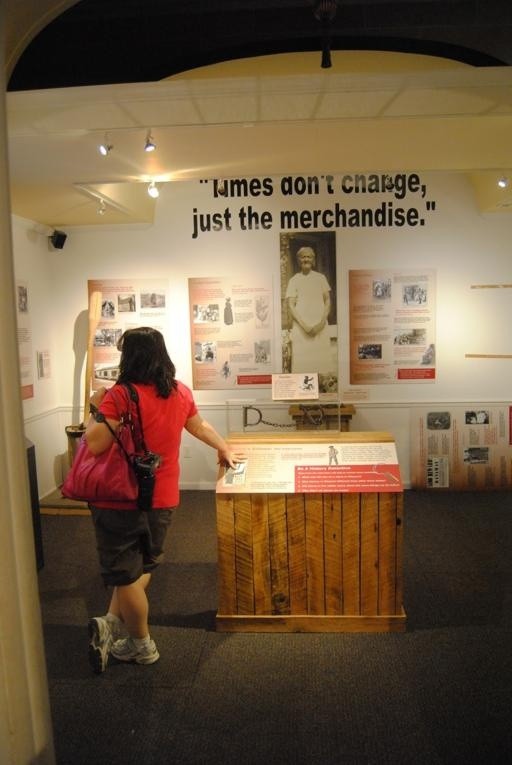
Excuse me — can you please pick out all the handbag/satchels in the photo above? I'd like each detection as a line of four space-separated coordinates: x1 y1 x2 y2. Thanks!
61 424 139 502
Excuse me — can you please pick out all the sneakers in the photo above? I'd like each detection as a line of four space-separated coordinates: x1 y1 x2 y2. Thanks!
111 636 160 665
87 616 114 674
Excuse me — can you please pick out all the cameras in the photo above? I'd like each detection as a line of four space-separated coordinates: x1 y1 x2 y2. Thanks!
134 452 160 513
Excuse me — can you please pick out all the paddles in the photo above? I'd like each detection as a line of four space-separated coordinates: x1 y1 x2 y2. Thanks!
83 291 103 428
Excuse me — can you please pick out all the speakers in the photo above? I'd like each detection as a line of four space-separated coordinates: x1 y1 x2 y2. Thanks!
49 229 67 248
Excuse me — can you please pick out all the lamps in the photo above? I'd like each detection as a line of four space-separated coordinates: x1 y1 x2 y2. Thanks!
149 181 160 198
98 132 113 157
143 129 156 151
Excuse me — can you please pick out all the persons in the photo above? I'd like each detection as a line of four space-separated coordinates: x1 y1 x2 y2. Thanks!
225 462 244 483
417 287 426 304
327 446 339 465
284 245 334 374
76 327 247 673
206 347 213 360
403 287 409 304
463 448 489 464
224 360 230 378
